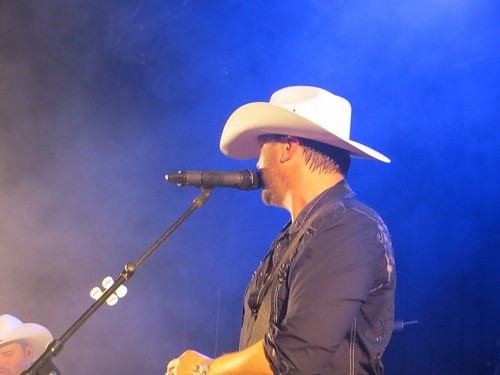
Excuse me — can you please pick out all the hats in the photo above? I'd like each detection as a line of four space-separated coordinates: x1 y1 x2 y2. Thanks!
0 314 55 363
219 83 391 167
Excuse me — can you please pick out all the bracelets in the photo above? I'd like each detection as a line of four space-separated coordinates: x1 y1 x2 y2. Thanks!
192 359 215 375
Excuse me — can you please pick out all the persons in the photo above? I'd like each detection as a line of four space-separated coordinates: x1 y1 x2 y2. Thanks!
162 85 397 375
0 314 54 375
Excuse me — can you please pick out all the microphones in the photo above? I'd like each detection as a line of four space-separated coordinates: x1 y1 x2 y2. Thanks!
164 169 264 190
